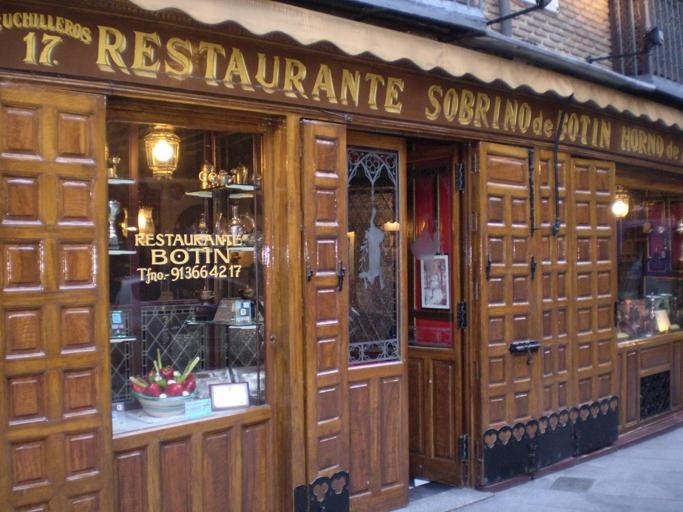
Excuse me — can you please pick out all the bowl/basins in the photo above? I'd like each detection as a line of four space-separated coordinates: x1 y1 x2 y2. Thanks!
131 387 199 419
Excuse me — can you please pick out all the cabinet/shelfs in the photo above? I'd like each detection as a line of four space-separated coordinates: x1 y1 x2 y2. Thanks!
107 178 136 344
185 183 265 371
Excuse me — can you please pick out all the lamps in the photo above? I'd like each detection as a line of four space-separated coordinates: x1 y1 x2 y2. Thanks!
122 205 155 243
586 27 666 64
616 184 630 218
488 1 551 27
143 125 180 181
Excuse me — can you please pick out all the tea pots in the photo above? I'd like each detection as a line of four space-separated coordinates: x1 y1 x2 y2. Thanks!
194 287 216 307
198 159 249 191
238 286 257 300
